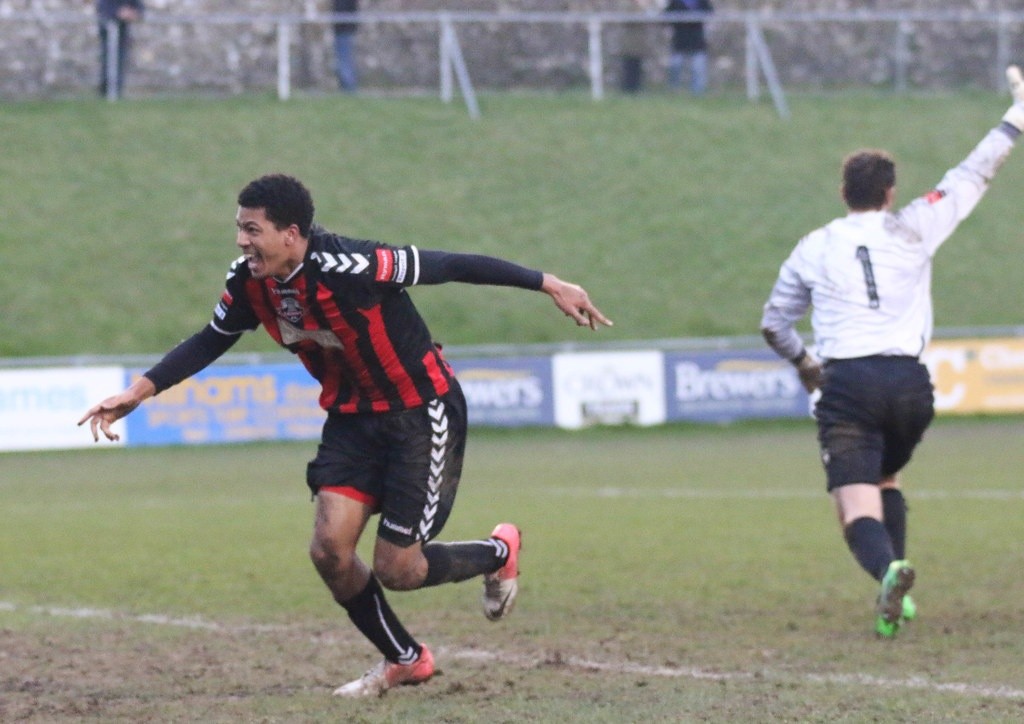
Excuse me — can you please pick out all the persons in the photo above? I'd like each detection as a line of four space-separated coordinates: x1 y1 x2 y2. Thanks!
79 173 613 698
757 65 1023 636
98 0 714 101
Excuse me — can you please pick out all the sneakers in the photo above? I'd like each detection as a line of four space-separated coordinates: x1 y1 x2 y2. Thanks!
332 642 434 699
875 593 916 622
481 523 522 621
873 559 916 640
